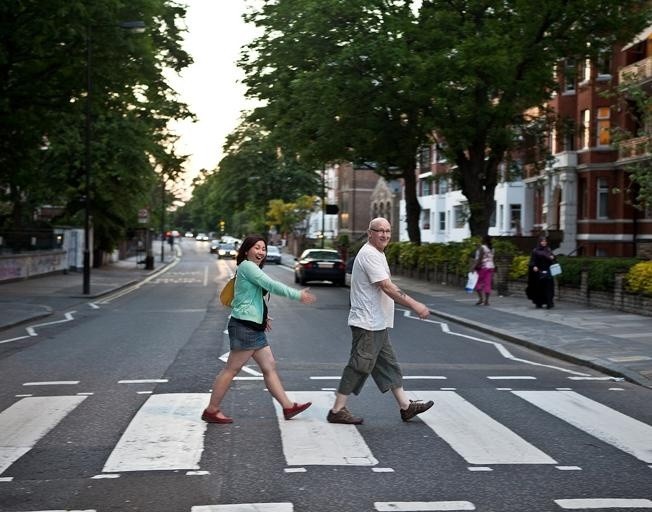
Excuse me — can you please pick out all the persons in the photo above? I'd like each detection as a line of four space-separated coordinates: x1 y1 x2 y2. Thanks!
327 216 435 424
201 236 317 424
525 236 557 308
470 235 495 306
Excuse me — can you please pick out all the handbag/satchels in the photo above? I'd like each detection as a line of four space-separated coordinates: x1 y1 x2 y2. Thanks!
549 263 562 277
219 277 236 308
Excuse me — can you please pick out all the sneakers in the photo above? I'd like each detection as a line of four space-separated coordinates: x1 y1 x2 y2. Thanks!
400 400 434 421
326 406 364 424
535 303 555 309
476 298 491 306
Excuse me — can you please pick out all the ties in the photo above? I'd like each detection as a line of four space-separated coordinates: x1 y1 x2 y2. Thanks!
283 402 312 420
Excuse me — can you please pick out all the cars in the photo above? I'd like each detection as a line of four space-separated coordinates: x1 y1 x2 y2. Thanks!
293 248 346 288
166 230 244 260
266 246 281 264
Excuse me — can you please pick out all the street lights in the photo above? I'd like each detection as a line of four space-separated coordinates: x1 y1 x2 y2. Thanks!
159 167 179 262
81 17 148 295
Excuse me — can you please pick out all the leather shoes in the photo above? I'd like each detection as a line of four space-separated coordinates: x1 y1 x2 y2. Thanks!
201 409 233 424
